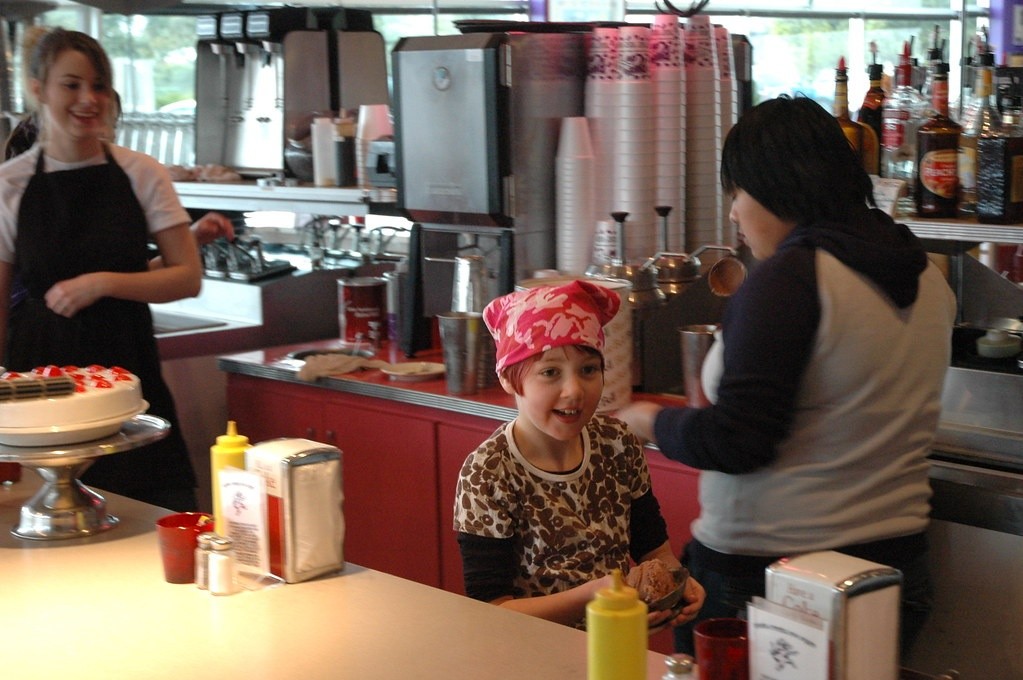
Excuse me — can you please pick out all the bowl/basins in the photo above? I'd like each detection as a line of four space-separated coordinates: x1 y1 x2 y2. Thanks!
647 568 689 629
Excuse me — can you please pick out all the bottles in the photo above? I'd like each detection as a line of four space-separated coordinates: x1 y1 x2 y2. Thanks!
585 567 647 680
209 420 253 537
309 106 356 187
828 0 1023 228
661 653 699 680
208 538 233 596
193 533 218 589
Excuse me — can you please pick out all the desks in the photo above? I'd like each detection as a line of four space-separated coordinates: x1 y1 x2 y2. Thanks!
0 479 699 680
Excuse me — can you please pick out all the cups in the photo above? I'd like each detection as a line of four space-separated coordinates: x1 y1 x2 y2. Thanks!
433 253 496 394
511 11 754 279
692 618 750 680
678 325 717 410
357 103 397 188
155 512 213 583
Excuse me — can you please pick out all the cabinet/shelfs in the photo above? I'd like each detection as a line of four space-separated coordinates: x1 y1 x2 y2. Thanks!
226 370 698 656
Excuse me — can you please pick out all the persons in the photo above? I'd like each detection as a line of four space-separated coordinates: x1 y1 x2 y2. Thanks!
618 92 960 659
1 26 233 513
449 280 706 635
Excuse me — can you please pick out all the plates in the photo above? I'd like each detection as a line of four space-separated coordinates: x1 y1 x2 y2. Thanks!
378 361 445 382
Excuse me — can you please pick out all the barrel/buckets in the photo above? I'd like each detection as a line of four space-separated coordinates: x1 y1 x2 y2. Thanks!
336 271 410 348
512 275 634 412
